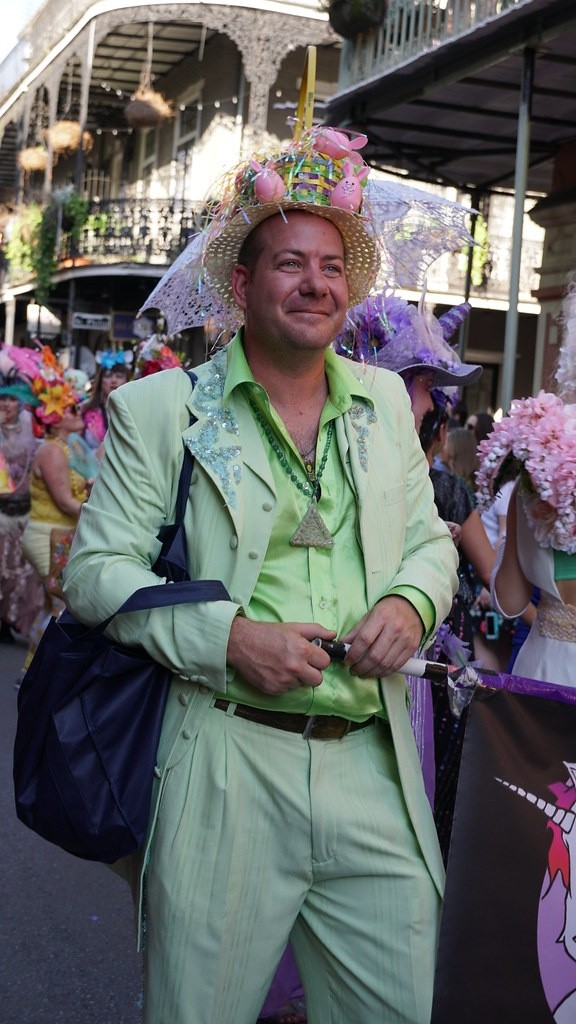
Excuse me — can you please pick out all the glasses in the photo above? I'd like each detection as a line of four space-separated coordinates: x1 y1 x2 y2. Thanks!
64 404 82 417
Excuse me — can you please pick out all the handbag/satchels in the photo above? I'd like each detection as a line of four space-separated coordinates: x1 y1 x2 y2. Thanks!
10 564 170 866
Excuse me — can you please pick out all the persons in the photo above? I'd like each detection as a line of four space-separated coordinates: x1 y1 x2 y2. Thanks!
1 192 575 1023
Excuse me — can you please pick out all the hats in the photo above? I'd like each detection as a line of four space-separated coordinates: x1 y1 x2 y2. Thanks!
207 151 380 312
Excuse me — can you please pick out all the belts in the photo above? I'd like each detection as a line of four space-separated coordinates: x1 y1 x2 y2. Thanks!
213 699 382 740
